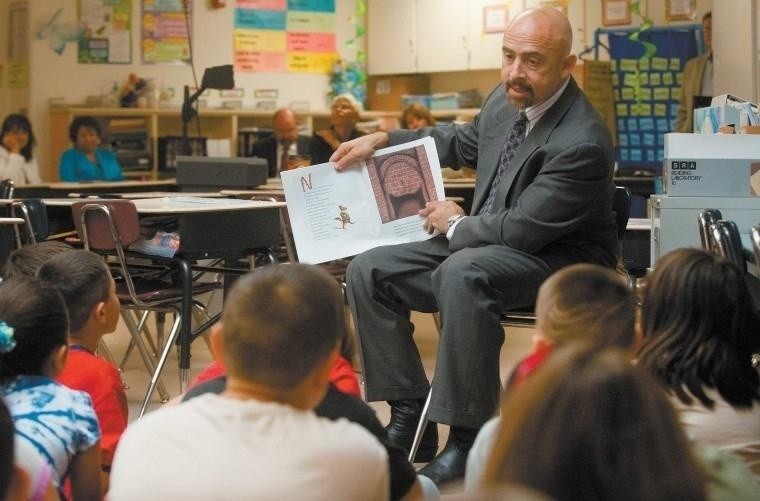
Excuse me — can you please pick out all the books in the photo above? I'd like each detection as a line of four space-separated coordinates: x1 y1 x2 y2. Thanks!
280 136 446 265
400 88 482 111
101 118 310 171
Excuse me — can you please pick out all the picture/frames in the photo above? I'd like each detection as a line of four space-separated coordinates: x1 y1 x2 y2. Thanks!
664 0 696 22
485 6 508 33
601 0 632 26
540 1 568 17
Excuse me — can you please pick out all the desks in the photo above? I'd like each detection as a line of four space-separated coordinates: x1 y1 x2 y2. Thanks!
609 173 662 198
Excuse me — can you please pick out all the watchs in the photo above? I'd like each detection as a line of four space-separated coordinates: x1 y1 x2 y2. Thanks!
445 214 464 235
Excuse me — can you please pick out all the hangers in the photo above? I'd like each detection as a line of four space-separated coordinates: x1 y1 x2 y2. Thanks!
577 27 618 62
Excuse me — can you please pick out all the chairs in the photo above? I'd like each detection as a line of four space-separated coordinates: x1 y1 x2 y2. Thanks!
698 223 759 279
699 208 722 252
407 186 632 466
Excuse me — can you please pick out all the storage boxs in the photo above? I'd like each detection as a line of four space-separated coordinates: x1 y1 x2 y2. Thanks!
663 131 759 197
426 96 482 109
401 94 430 111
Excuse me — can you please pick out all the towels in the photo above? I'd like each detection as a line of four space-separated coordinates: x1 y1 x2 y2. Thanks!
583 58 618 148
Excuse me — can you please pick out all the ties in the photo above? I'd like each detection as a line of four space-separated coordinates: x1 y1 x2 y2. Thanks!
281 144 289 171
474 113 526 215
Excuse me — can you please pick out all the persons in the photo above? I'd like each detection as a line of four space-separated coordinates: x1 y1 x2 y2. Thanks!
311 94 370 165
0 114 40 186
102 261 441 501
0 242 129 501
328 6 622 491
466 247 760 501
400 103 436 130
60 116 124 181
252 109 310 178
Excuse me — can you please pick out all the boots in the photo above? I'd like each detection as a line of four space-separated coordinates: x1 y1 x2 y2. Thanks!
384 399 438 463
417 425 479 485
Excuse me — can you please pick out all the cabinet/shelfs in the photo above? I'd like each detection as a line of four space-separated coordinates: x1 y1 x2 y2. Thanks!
48 100 479 184
366 68 503 111
646 194 759 278
